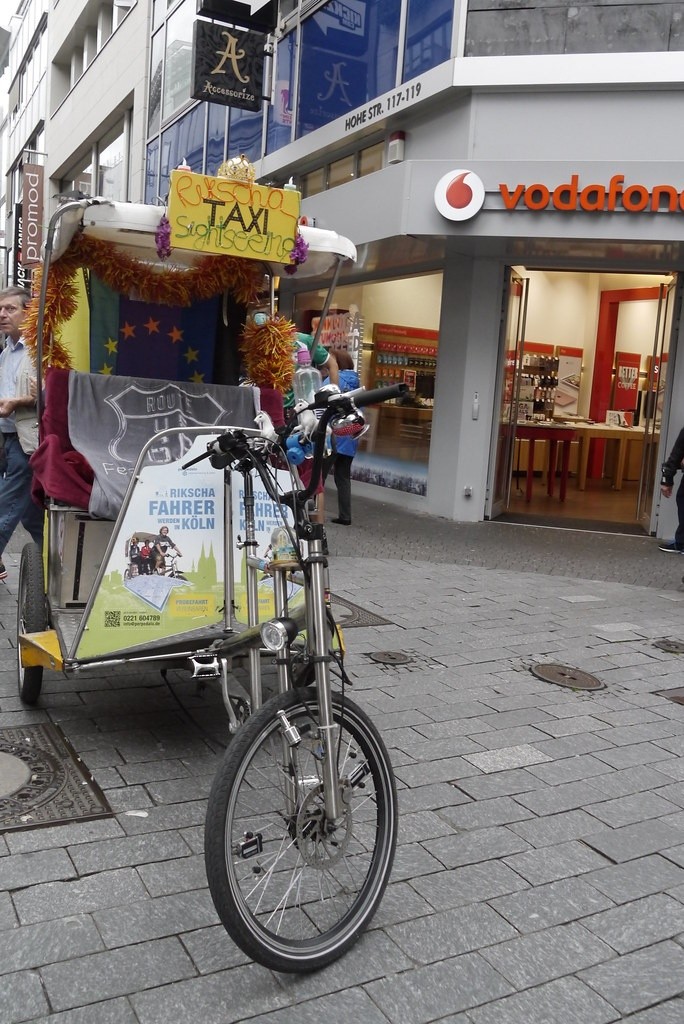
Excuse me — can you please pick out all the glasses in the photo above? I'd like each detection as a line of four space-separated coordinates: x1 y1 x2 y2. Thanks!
0 305 25 314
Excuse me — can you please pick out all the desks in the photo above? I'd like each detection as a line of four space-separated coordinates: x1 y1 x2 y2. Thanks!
498 419 660 505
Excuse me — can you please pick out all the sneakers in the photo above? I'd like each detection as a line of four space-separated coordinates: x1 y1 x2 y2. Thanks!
658 544 684 553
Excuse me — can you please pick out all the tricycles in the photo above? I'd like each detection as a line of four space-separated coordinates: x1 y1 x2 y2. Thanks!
18 198 409 974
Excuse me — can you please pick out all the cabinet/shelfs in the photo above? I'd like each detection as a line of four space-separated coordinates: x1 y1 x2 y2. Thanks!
519 345 562 420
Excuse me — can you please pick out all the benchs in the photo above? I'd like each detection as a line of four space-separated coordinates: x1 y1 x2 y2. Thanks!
34 363 291 518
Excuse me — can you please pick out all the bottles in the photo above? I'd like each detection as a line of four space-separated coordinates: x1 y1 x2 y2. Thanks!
293 349 322 405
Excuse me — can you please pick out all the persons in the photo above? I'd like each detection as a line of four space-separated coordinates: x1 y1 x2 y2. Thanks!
129 526 183 579
0 287 48 579
245 310 361 526
659 426 684 554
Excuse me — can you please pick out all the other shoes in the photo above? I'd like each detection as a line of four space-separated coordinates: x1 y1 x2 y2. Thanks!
0 565 7 578
332 519 352 526
